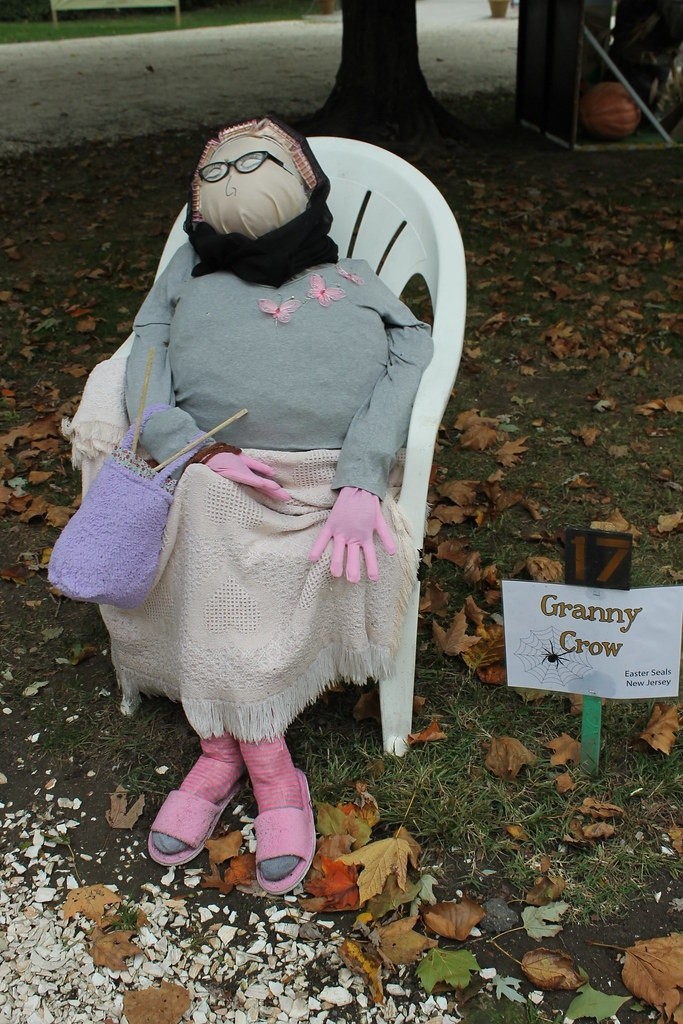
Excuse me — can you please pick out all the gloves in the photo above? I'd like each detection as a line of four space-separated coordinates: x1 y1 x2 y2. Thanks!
198 447 291 501
314 487 396 583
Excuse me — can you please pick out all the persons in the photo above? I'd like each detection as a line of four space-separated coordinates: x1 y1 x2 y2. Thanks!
119 113 433 894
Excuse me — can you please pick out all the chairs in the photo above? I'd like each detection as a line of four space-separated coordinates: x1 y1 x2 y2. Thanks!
62 135 468 756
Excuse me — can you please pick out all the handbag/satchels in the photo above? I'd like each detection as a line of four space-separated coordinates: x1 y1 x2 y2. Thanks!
47 403 213 611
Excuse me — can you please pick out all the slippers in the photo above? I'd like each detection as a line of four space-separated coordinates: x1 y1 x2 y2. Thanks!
147 768 249 866
252 768 316 895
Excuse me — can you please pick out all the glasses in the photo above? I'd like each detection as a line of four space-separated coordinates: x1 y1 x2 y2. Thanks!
197 151 304 185
